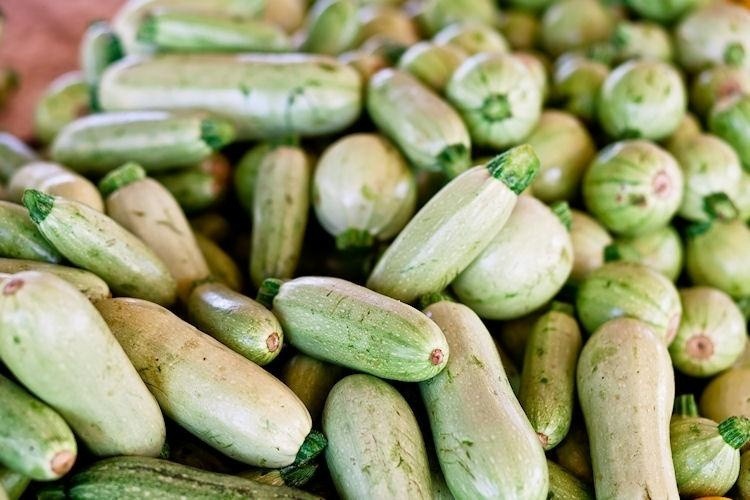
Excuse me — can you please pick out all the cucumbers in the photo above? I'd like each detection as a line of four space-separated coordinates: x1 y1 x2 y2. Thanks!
1 0 750 500
